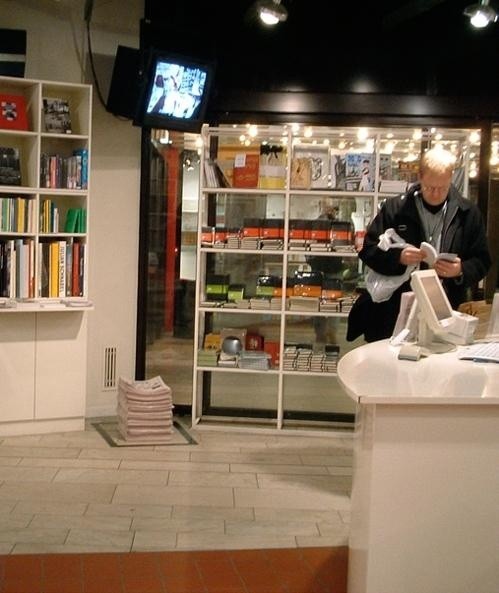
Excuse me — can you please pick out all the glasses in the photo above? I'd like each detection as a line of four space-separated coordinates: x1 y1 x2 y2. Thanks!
422 185 448 194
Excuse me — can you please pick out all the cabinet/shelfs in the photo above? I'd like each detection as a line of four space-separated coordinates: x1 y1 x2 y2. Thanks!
191 122 482 427
1 70 98 435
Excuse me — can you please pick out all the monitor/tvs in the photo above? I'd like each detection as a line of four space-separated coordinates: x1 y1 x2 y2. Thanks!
106 44 221 133
410 269 456 355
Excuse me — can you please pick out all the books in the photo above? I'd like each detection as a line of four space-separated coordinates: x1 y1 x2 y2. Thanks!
195 137 463 372
114 372 174 441
1 88 89 309
459 339 499 363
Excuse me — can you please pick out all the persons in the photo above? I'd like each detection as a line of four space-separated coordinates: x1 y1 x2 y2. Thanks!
359 145 493 341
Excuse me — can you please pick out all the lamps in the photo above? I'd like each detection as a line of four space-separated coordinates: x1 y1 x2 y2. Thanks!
259 0 288 35
464 0 498 33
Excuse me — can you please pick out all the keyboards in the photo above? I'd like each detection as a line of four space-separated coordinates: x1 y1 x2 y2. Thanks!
458 343 499 363
